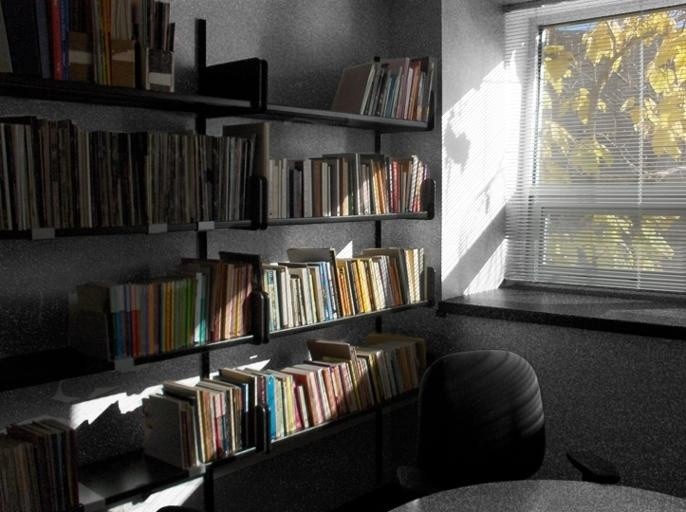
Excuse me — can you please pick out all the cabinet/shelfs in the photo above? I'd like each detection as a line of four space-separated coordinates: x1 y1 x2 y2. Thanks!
0 2 442 512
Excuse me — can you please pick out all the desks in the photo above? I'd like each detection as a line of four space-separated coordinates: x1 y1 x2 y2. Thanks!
387 479 686 511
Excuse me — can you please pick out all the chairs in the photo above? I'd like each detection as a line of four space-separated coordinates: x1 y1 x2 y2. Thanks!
419 350 624 485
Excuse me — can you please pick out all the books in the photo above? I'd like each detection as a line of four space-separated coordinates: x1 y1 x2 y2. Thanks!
142 368 259 471
0 0 176 91
236 336 432 442
0 117 253 230
76 262 256 361
218 245 429 332
222 122 430 219
331 55 432 122
0 419 81 512
